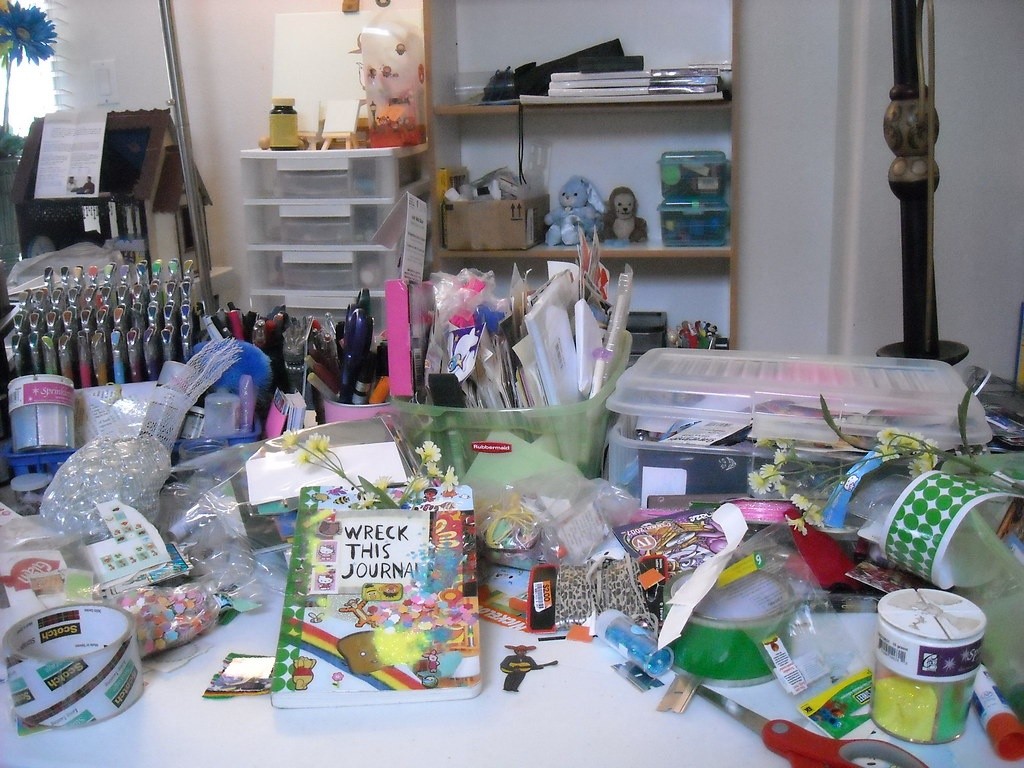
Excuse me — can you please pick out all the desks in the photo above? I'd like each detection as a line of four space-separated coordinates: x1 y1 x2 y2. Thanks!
0 552 1024 768
192 265 235 310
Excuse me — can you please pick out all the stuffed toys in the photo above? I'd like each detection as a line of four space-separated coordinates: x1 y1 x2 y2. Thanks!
544 175 606 247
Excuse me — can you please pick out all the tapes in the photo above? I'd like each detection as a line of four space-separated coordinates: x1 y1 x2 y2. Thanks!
658 566 794 688
1 603 144 731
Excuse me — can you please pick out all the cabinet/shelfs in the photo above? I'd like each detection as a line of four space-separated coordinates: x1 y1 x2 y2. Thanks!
241 138 430 336
423 0 739 348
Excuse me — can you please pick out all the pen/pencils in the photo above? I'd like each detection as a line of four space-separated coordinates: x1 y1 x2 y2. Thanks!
199 298 292 364
304 288 391 409
10 258 196 386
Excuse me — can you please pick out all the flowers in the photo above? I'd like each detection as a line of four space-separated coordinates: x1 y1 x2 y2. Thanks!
280 425 460 510
0 0 58 156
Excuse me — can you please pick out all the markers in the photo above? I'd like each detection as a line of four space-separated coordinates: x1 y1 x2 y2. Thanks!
971 661 1024 762
339 310 374 405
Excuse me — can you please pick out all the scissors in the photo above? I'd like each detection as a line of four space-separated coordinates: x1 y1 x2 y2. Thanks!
696 685 930 768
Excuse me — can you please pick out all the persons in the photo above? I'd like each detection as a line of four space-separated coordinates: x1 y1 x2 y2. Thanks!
595 186 648 243
67 176 95 194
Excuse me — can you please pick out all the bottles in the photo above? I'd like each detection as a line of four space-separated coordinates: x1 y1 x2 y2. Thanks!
269 98 299 151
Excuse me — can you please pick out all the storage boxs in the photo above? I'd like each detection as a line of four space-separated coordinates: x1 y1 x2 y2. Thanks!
604 347 992 499
393 329 632 479
444 194 550 251
656 150 726 204
656 201 731 247
